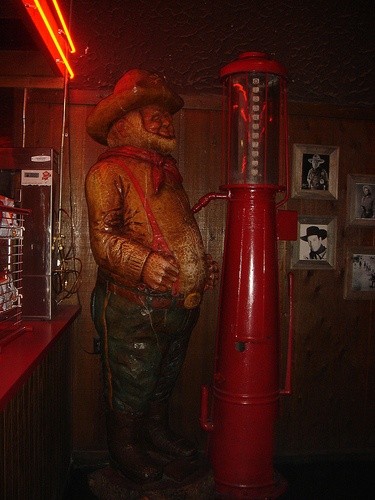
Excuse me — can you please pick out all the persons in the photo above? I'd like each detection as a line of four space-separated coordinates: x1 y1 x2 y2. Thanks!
300 226 328 261
85 69 219 483
360 186 374 218
308 154 329 192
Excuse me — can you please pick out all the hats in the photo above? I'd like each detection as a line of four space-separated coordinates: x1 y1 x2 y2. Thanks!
300 226 327 242
308 154 325 164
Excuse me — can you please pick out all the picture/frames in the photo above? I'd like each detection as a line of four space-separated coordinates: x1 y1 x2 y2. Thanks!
345 173 375 229
342 246 375 302
291 215 337 270
292 143 340 200
